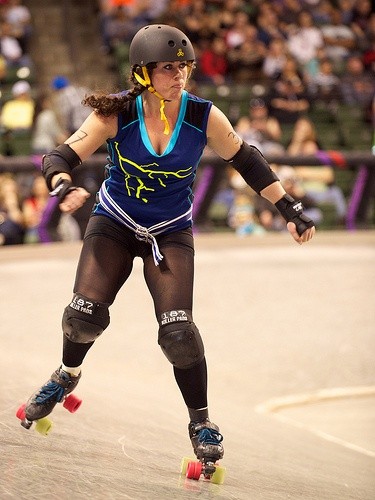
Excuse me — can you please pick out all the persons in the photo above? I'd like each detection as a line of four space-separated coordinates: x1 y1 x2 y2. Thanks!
1 0 375 248
15 24 316 486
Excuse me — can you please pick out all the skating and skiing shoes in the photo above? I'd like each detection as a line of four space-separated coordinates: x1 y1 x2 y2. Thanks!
178 419 225 486
14 366 85 435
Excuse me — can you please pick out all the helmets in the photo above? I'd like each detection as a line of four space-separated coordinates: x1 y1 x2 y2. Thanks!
129 24 197 67
